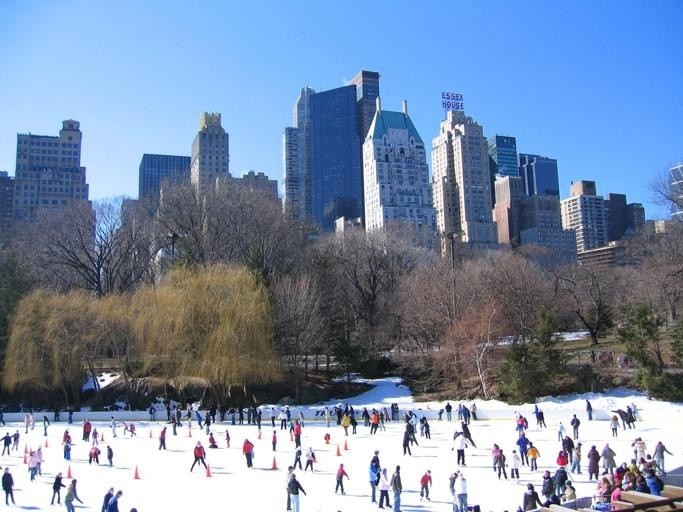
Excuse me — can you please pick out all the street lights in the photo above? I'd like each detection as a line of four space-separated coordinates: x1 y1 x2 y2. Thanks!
434 229 469 390
163 231 189 261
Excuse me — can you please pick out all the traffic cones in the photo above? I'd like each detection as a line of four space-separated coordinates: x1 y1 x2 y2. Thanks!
289 433 294 441
271 456 277 470
335 443 341 456
257 432 261 439
343 439 349 450
187 430 191 437
21 433 142 480
149 430 153 437
325 434 330 444
312 455 316 462
205 463 211 477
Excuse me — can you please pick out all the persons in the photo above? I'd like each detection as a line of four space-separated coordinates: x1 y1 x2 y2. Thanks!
587 346 631 371
490 399 673 511
362 401 481 511
123 420 137 438
1 406 122 512
266 403 358 511
147 403 263 473
